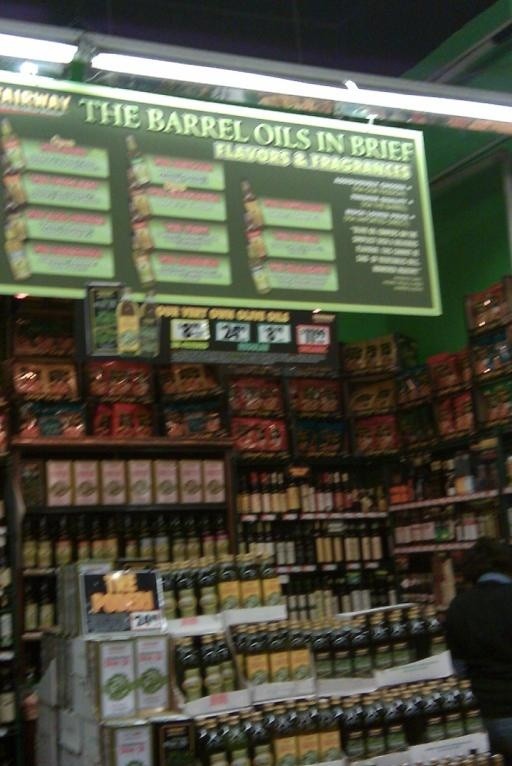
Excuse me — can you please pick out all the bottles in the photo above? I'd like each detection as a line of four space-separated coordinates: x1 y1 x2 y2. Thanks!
0 436 512 766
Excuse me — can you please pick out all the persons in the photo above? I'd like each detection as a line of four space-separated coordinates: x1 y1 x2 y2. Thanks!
446 536 511 761
